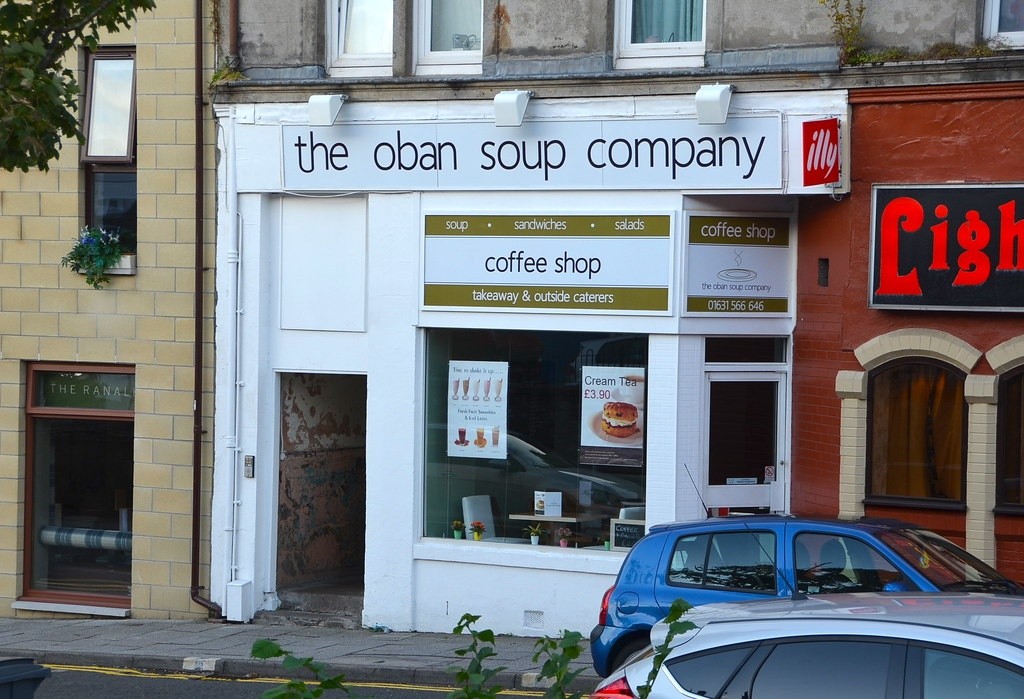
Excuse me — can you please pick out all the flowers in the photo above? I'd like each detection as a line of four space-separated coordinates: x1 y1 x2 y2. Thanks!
555 526 573 539
469 521 486 537
450 520 466 532
62 222 122 289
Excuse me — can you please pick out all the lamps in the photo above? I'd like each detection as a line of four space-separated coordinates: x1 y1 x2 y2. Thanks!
695 81 736 125
494 88 535 128
307 93 350 128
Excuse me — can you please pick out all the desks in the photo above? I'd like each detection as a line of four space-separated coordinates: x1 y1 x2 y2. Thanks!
508 512 586 546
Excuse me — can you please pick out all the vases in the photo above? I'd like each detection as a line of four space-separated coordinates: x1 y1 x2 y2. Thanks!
454 529 463 540
559 538 568 548
473 532 482 541
104 253 137 270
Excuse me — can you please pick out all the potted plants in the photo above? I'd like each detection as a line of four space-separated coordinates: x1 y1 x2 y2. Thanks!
521 521 547 545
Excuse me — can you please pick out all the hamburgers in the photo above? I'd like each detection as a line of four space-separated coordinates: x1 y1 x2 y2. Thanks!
601 402 641 438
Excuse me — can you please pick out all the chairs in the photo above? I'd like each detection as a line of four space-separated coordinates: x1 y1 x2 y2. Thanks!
583 507 646 551
461 494 532 544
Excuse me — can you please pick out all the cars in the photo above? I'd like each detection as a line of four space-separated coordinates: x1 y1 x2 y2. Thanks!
590 588 1024 699
427 421 646 546
589 461 1024 679
